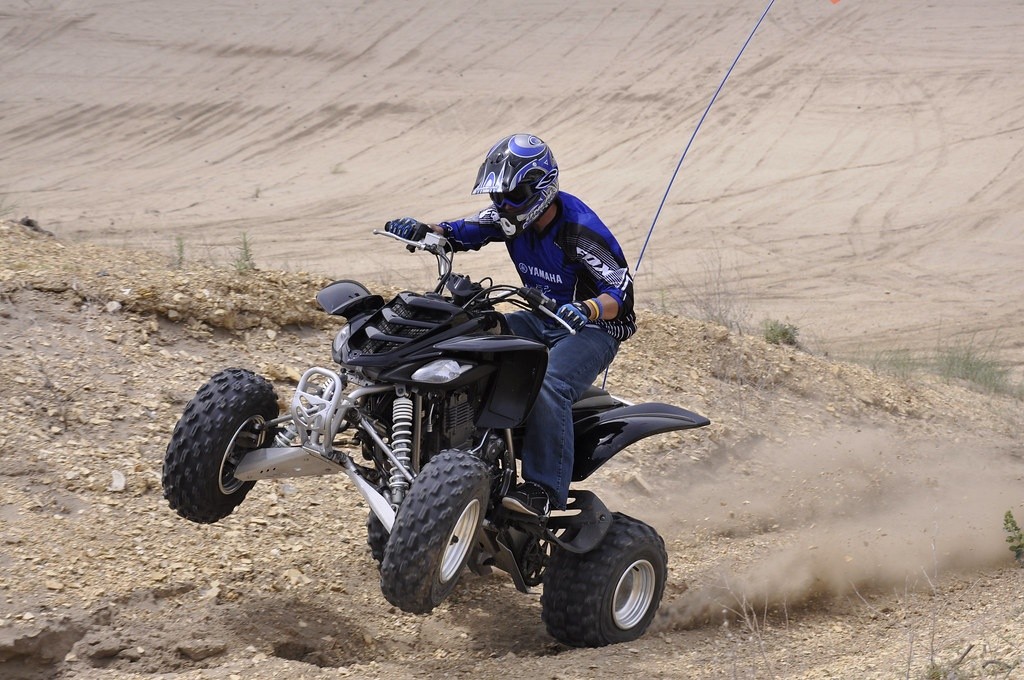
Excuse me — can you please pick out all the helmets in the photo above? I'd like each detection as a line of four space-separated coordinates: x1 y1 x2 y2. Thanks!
471 131 559 240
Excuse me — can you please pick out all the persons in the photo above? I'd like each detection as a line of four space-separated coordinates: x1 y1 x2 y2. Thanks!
384 134 638 517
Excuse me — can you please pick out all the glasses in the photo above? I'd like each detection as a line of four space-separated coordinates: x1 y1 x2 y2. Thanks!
489 178 543 208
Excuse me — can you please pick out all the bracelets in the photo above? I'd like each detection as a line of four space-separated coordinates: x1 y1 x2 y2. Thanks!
588 298 602 320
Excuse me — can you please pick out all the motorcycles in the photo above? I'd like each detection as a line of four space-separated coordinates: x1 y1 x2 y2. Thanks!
162 222 711 646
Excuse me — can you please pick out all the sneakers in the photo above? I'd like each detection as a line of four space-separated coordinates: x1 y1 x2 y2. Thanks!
501 481 551 517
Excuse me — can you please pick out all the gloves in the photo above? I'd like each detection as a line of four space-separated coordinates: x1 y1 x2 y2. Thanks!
555 301 590 335
385 217 431 242
